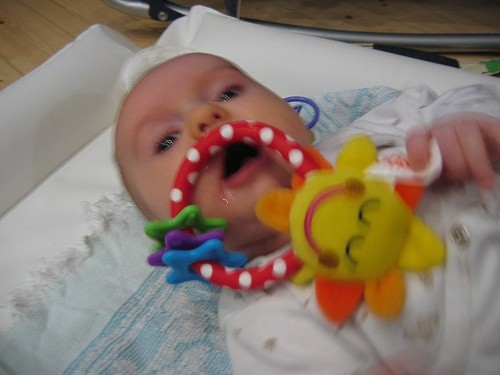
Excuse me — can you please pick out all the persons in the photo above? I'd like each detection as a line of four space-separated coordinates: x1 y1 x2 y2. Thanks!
114 51 499 375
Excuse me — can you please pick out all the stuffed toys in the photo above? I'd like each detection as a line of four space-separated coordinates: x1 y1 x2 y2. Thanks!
254 133 447 325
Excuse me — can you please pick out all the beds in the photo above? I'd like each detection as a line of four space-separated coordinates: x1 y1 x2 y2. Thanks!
0 4 500 375
